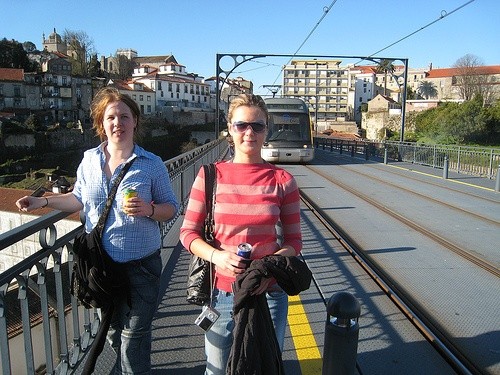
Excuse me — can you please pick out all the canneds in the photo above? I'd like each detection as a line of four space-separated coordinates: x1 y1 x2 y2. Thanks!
237 243 252 269
123 187 138 213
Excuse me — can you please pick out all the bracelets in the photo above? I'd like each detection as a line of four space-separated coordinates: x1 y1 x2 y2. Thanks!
42 197 48 208
146 201 155 218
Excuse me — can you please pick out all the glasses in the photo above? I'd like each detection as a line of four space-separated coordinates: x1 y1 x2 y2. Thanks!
229 121 269 135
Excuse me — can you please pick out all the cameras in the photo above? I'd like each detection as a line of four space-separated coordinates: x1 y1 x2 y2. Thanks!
194 306 220 332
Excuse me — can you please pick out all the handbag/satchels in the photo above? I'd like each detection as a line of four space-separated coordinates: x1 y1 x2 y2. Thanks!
69 229 118 310
185 231 217 306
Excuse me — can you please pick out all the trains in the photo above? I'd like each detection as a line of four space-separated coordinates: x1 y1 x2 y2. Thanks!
261 97 314 163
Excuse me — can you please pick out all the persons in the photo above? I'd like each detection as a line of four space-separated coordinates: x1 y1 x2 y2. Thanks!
15 88 179 375
179 93 302 375
